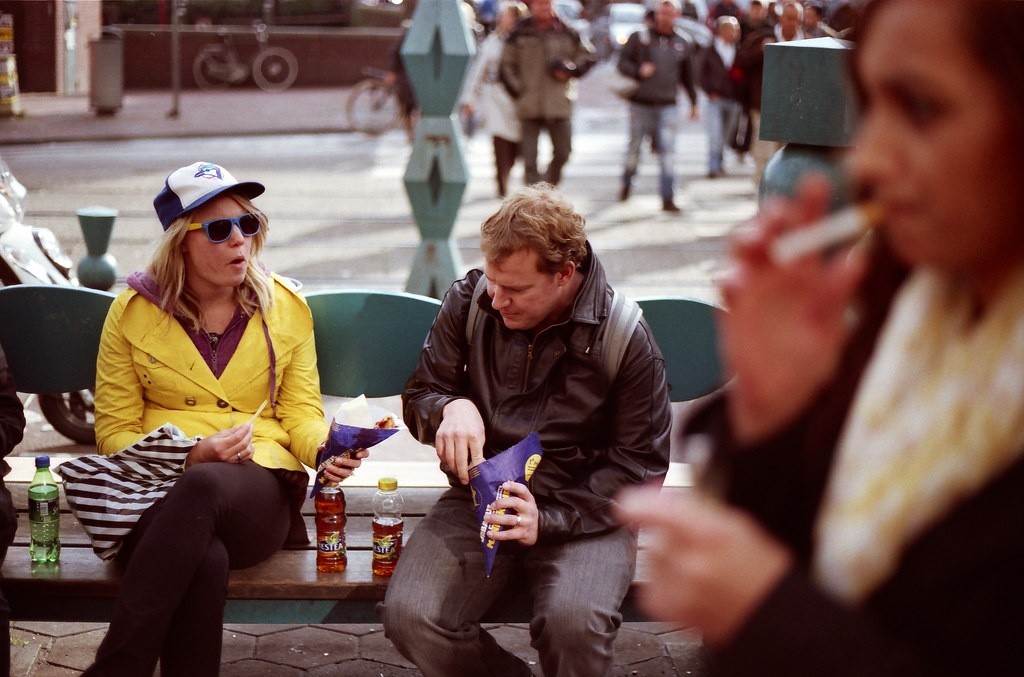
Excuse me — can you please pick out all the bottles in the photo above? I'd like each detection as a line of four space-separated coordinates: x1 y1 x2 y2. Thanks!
371 477 405 578
27 455 61 564
314 481 347 574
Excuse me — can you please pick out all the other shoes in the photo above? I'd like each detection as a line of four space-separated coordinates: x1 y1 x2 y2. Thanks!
620 175 632 199
663 202 679 212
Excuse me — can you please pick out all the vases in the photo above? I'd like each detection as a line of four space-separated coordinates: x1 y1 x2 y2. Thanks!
76 205 120 288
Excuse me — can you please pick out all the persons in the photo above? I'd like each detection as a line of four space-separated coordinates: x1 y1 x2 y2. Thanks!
79 163 368 677
0 344 27 677
613 0 1024 677
384 0 853 213
381 181 673 677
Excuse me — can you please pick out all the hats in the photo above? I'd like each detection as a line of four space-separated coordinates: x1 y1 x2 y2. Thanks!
154 161 266 232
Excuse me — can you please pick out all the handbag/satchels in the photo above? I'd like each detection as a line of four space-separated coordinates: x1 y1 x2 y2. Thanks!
52 422 203 561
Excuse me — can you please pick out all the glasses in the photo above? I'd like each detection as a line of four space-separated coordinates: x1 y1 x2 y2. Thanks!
186 211 261 242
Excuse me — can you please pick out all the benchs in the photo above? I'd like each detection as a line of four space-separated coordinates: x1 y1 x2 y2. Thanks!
0 265 731 623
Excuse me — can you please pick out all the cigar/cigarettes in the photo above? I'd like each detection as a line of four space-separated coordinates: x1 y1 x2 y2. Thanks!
772 197 890 269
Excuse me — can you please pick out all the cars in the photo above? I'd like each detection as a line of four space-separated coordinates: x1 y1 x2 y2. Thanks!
591 2 650 57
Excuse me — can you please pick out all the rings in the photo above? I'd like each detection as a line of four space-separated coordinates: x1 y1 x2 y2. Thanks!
515 515 520 527
237 452 242 460
346 469 353 477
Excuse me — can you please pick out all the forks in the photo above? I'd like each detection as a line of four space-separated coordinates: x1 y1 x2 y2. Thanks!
249 399 268 424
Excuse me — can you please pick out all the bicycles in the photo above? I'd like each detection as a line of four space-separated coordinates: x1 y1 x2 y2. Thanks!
0 159 97 447
192 17 299 94
340 63 479 140
